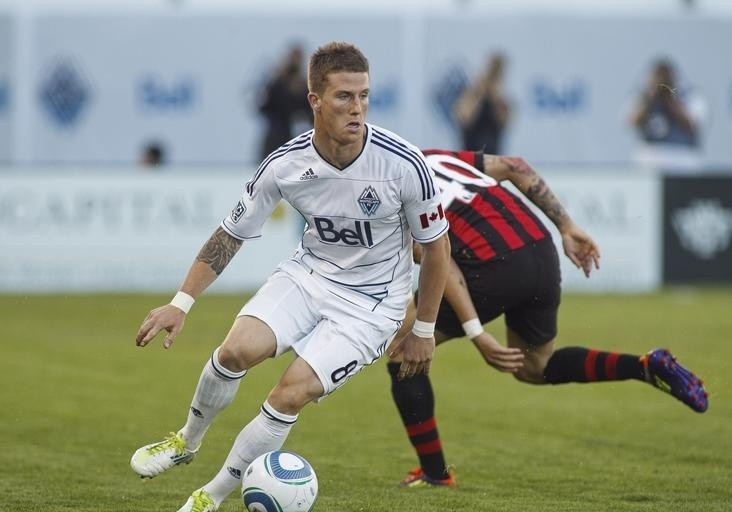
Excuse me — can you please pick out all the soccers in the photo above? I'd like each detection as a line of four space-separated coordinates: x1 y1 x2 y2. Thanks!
241 451 318 512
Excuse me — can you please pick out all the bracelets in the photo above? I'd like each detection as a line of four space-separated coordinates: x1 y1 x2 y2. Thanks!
411 318 436 339
170 290 196 315
461 317 485 341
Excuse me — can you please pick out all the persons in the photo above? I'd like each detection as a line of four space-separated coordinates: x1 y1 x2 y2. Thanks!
453 53 512 153
631 60 697 143
127 37 454 511
258 48 316 164
372 144 712 490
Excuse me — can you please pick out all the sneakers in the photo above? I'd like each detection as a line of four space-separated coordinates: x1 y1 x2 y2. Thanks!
130 432 202 479
176 486 222 512
639 347 710 414
400 464 457 490
